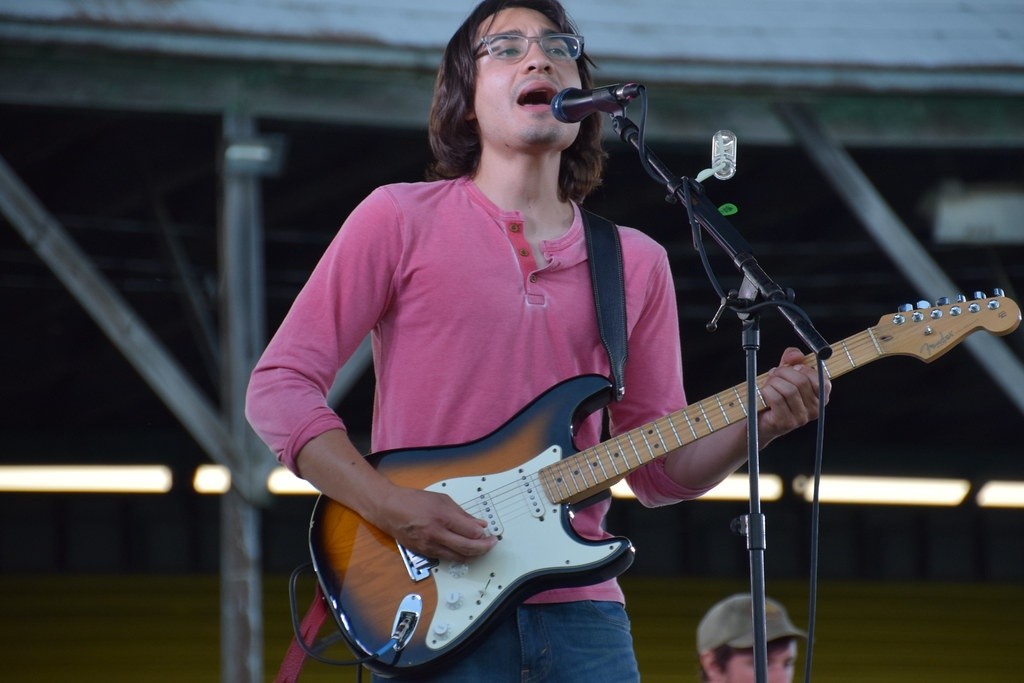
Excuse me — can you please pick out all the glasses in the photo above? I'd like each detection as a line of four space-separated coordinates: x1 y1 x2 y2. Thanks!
472 32 584 61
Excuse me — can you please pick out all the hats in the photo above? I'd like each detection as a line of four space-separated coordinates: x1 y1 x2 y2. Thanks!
695 593 807 655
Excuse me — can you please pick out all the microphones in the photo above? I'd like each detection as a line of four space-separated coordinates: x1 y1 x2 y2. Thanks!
551 82 646 123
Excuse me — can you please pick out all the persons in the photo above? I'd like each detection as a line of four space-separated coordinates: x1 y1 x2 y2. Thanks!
244 0 832 683
696 594 807 683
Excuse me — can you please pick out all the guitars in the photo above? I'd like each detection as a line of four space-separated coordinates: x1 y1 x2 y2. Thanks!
305 282 1022 683
